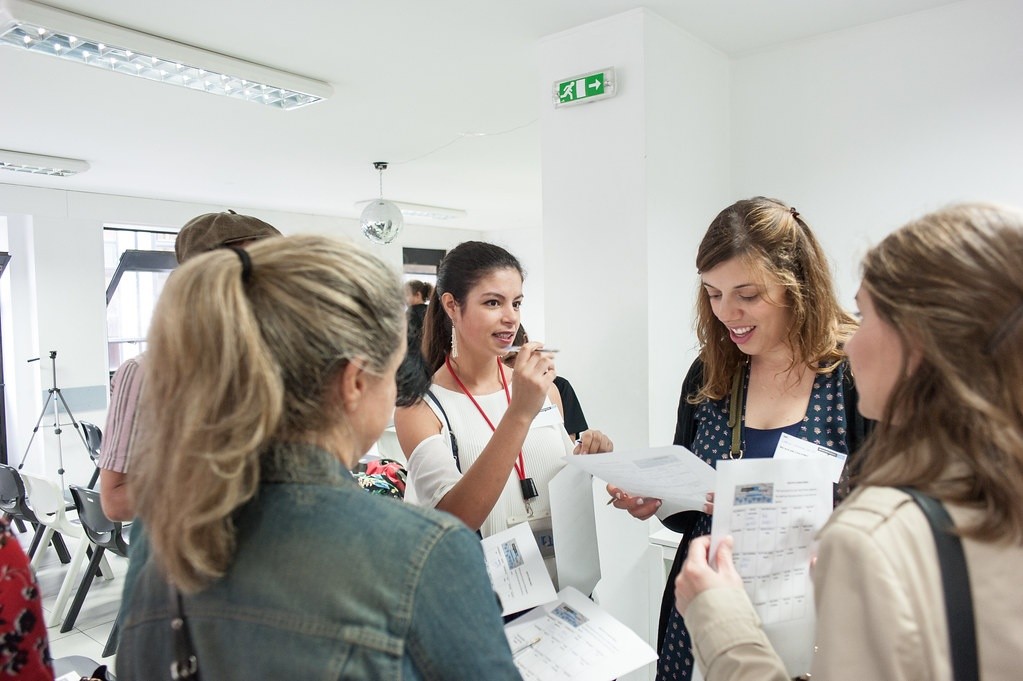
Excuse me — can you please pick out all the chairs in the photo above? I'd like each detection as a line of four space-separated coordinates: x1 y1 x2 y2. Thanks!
0 463 71 569
20 469 114 626
58 483 136 659
79 419 102 489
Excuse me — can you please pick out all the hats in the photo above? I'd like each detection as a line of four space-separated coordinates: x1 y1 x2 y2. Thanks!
175 209 283 267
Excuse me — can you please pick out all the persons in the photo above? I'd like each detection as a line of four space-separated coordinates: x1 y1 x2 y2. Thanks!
500 323 588 444
607 197 873 681
113 236 520 681
0 515 55 681
397 241 611 588
97 211 285 521
402 279 434 348
677 202 1023 681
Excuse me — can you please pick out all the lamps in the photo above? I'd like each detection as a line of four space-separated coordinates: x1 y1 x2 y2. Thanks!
0 0 328 113
0 149 91 178
361 162 404 247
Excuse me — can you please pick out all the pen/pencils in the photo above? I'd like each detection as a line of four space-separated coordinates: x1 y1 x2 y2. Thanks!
606 495 617 505
502 345 561 353
512 639 541 660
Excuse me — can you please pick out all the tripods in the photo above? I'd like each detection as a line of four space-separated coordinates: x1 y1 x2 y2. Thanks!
16 350 99 499
